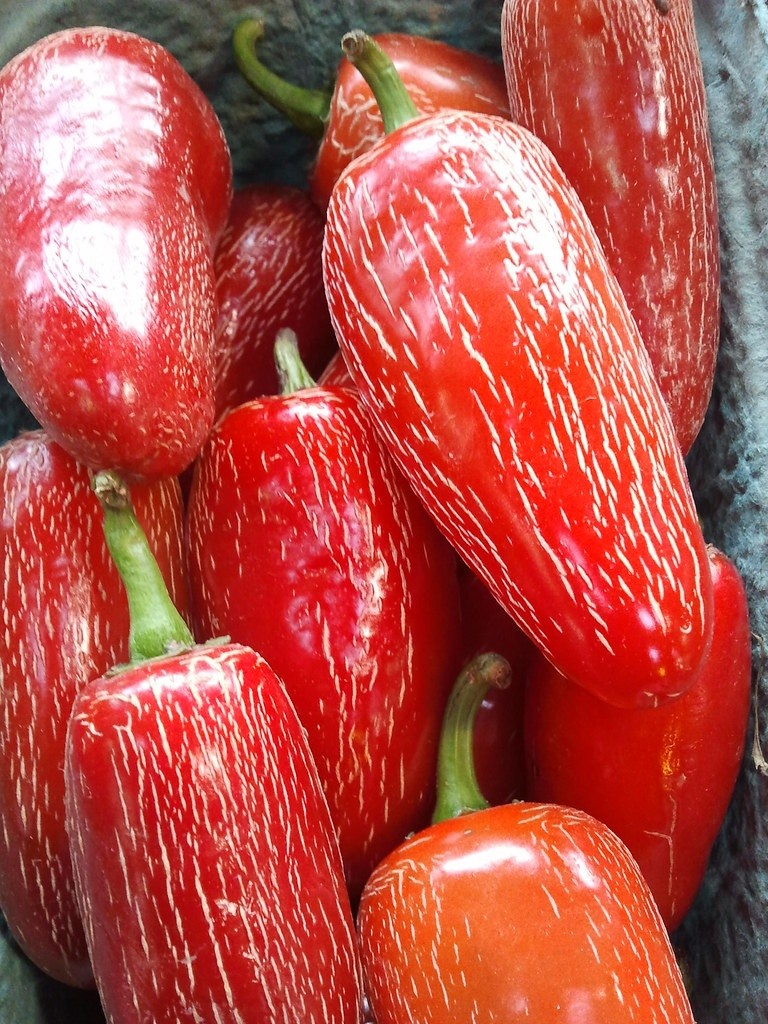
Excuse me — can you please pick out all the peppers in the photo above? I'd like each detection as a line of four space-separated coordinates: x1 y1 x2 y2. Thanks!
2 1 767 1024
